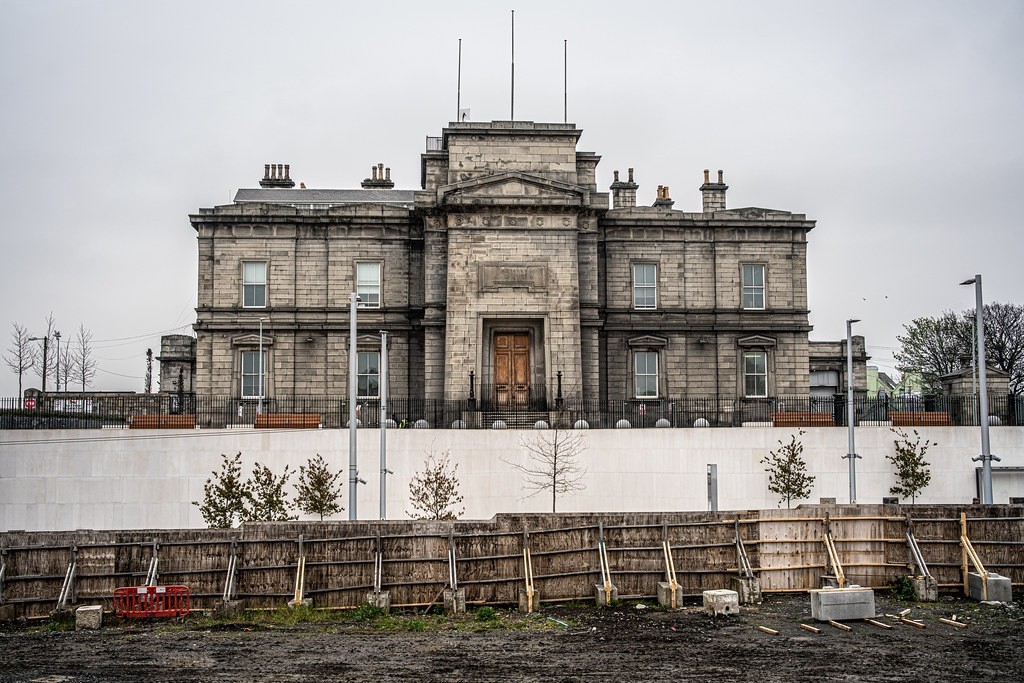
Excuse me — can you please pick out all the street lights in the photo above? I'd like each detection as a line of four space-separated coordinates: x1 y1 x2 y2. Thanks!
55 332 63 393
28 335 47 390
348 291 363 521
841 320 863 505
258 317 267 414
968 315 978 427
958 274 1001 504
379 330 390 522
